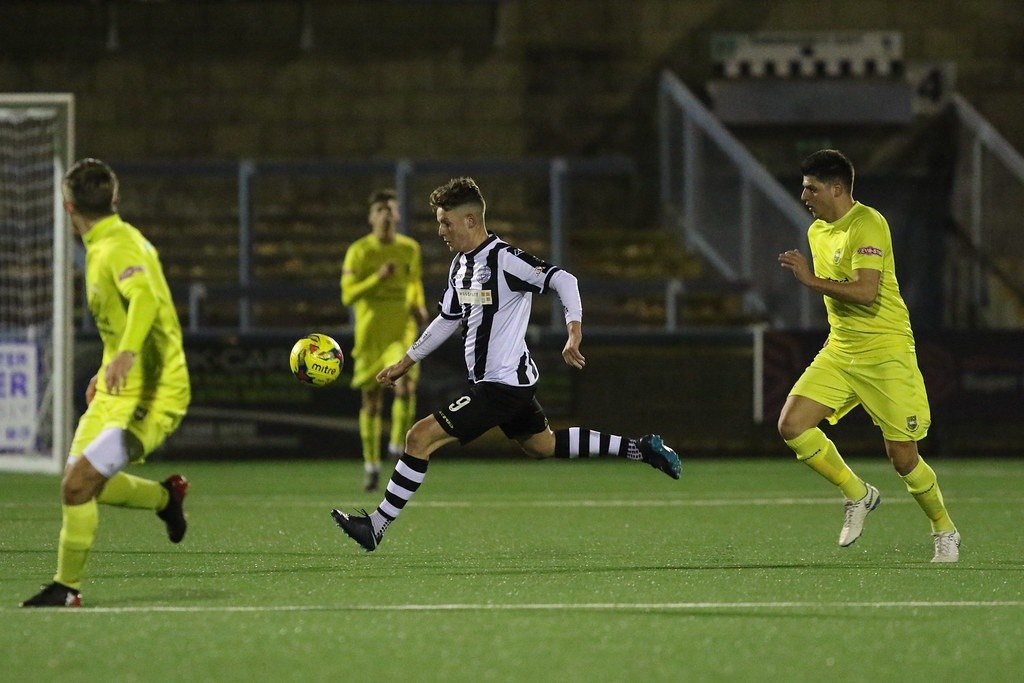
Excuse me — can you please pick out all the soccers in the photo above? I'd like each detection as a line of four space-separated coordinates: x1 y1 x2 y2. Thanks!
289 333 344 388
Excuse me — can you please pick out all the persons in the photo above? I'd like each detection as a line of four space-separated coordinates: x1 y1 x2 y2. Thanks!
340 190 428 491
330 179 682 552
777 150 962 563
18 158 191 609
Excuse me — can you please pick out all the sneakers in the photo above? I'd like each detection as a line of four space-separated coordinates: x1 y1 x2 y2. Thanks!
17 581 82 608
635 433 683 480
155 475 189 543
930 527 961 563
838 482 880 547
330 507 384 552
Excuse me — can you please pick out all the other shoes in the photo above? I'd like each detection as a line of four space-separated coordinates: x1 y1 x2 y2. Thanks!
365 470 380 492
389 451 402 464
